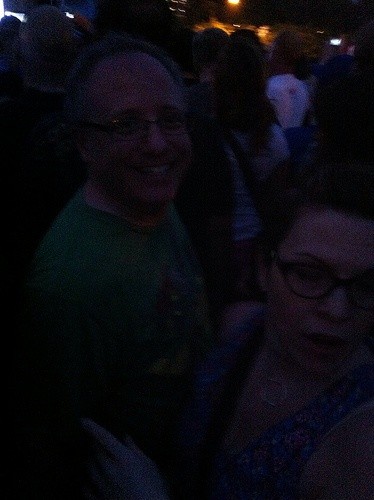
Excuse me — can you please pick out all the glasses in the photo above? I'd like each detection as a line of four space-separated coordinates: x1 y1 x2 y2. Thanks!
89 114 191 143
275 253 374 310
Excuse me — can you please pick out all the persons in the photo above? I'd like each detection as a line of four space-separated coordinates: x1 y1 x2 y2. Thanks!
27 38 233 500
1 2 374 171
192 143 373 500
